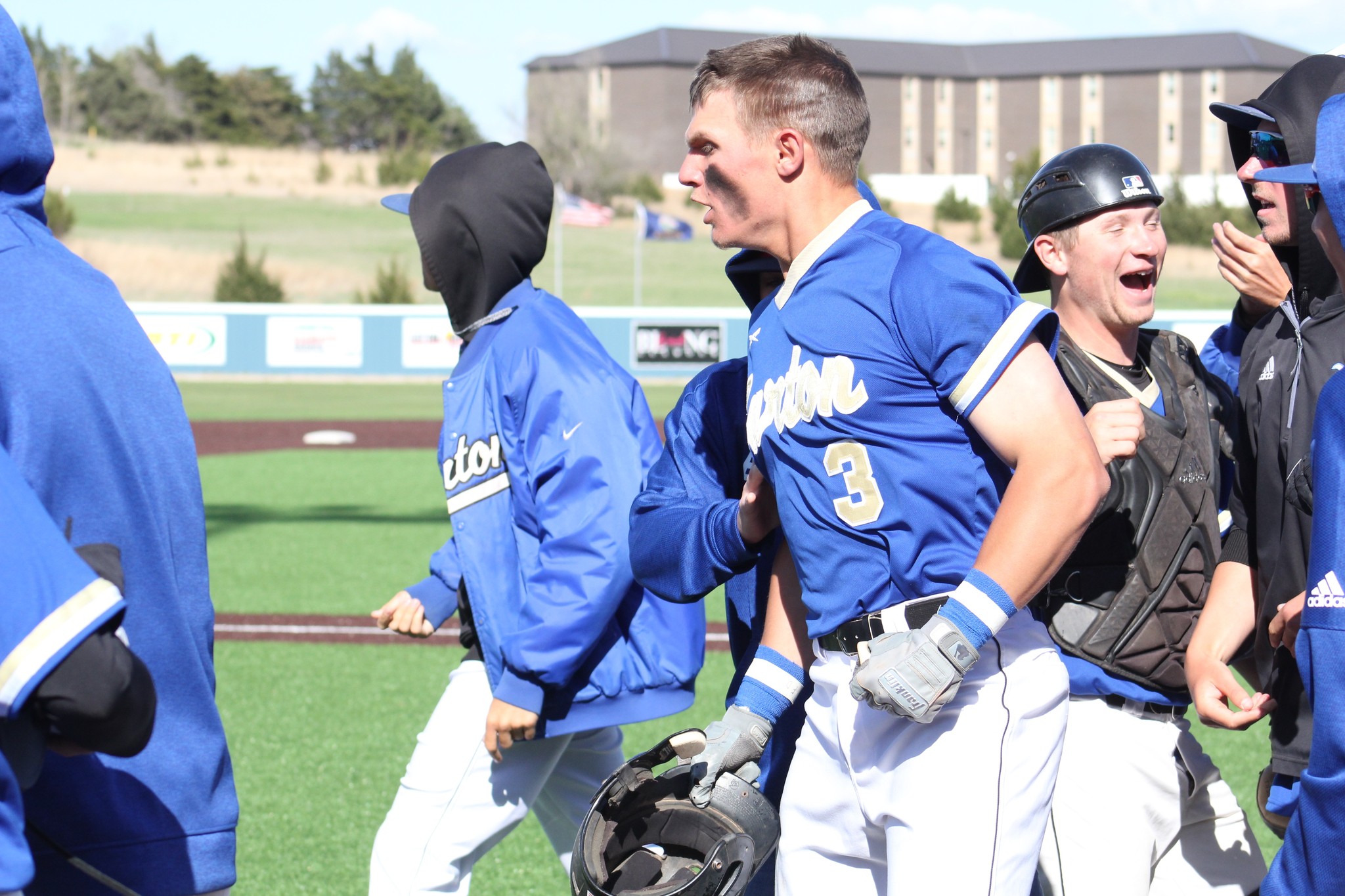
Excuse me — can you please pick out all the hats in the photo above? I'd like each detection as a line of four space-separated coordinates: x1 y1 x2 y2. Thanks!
380 193 411 215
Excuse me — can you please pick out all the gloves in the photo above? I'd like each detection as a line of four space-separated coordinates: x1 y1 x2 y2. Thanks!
676 702 773 808
849 613 980 729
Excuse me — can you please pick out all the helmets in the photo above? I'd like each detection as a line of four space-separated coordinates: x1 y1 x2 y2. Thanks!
1012 142 1165 293
565 727 782 895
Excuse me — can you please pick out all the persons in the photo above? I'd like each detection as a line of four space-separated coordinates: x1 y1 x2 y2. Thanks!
0 0 240 896
629 54 1345 896
367 140 708 896
675 33 1115 896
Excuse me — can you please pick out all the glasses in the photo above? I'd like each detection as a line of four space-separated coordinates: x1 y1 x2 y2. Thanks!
1245 130 1289 164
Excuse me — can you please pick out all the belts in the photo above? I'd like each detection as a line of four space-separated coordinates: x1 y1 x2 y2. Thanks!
817 595 950 657
1107 693 1188 716
